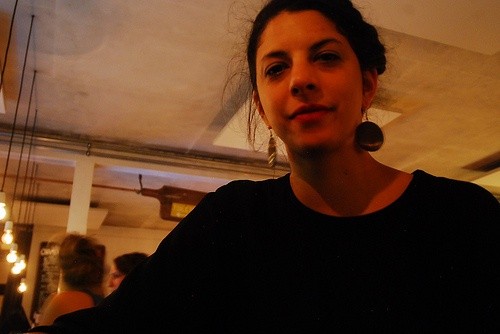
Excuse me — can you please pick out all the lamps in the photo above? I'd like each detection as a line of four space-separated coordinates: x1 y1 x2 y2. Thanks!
1 0 38 294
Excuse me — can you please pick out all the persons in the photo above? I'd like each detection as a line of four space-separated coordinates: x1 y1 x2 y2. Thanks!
30 233 107 325
39 0 500 334
109 250 149 294
0 284 31 334
